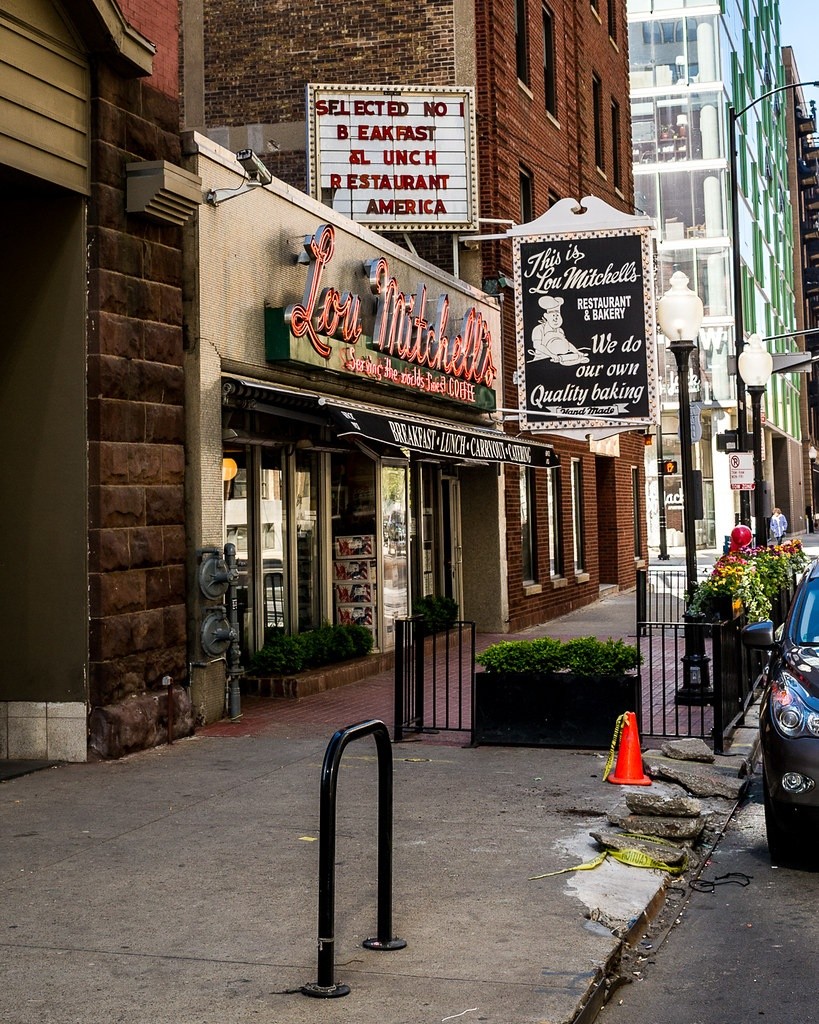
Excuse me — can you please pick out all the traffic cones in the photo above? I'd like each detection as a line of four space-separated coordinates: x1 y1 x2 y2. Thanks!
607 712 653 787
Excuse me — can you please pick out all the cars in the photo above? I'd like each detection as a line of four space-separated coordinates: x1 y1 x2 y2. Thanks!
741 556 819 867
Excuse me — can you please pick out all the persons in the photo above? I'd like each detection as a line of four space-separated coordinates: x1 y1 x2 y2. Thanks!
805 504 814 533
770 508 787 545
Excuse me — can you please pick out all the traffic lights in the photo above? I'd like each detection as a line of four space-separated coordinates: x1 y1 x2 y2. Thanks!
662 459 677 475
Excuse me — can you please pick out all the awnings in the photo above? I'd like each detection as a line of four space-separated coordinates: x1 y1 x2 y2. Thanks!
228 382 563 476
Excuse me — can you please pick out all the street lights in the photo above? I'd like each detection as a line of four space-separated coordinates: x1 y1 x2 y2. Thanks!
729 81 819 548
644 424 670 560
808 446 818 519
657 271 714 706
734 333 780 542
